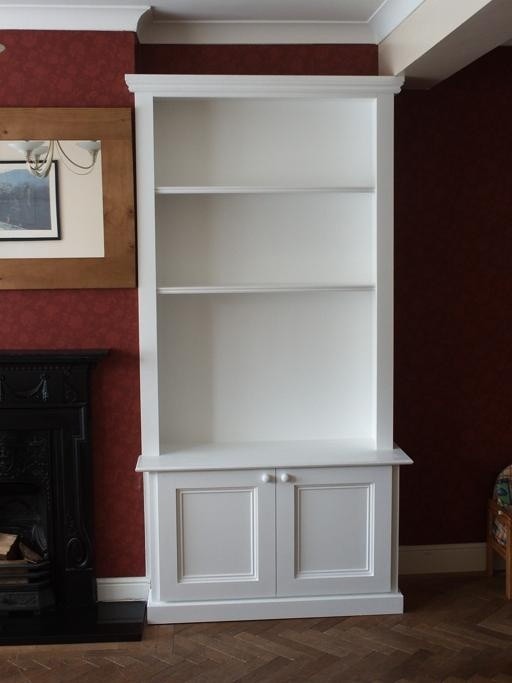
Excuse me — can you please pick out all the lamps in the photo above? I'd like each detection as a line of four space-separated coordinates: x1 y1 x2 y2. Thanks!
7 139 100 179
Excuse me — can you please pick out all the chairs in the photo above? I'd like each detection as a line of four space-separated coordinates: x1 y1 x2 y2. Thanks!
486 465 511 600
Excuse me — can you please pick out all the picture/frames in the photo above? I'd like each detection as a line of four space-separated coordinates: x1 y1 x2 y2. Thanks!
1 160 60 242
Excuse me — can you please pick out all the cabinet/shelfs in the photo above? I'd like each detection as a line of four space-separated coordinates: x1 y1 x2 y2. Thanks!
124 72 415 623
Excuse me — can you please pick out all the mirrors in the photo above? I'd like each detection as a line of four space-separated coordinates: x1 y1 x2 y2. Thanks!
0 107 137 289
